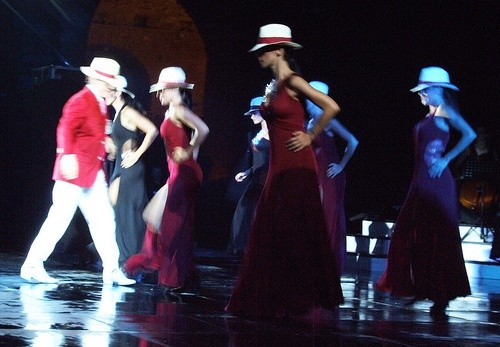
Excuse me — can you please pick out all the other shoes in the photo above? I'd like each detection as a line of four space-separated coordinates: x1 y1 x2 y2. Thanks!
20 262 59 284
103 269 136 286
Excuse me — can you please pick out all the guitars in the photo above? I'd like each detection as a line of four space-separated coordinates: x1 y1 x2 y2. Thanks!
457 132 495 209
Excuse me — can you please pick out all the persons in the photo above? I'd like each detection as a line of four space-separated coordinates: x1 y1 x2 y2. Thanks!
304 80 359 270
122 67 210 295
221 97 270 256
20 57 136 286
106 78 159 279
224 24 344 319
377 66 500 317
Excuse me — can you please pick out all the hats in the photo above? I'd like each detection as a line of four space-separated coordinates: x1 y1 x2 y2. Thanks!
309 81 330 97
411 66 459 94
118 75 135 98
245 96 263 117
148 67 194 92
249 23 301 51
78 57 122 90
